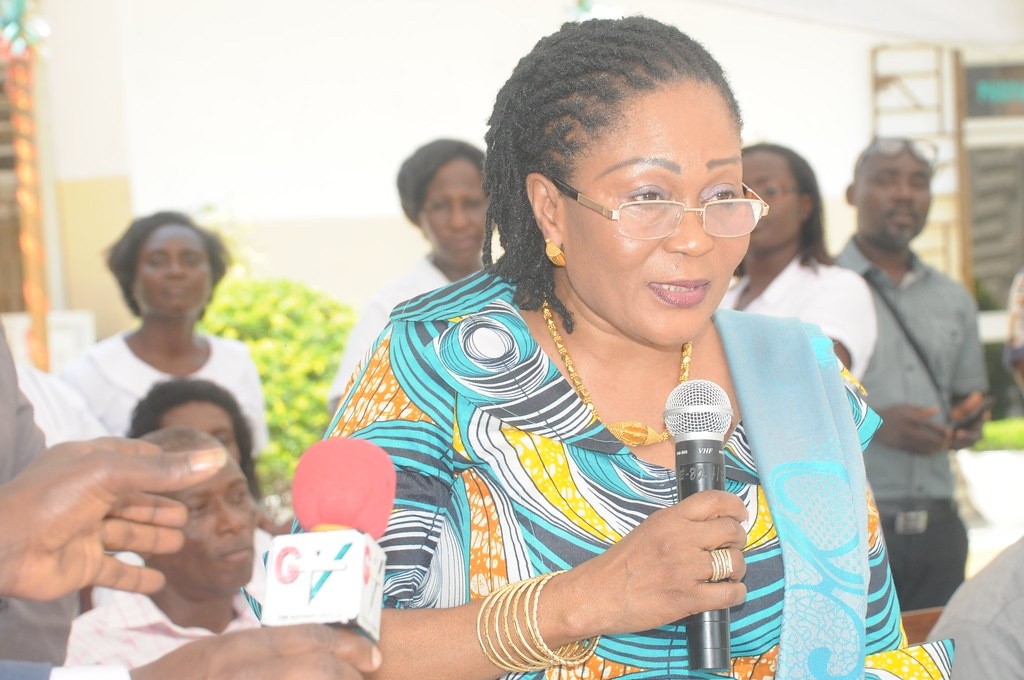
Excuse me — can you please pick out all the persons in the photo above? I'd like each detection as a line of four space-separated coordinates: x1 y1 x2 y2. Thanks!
925 269 1024 680
0 436 229 603
124 379 303 536
288 15 955 680
83 211 268 455
0 320 78 679
831 139 991 611
719 142 879 384
64 426 386 680
328 139 496 415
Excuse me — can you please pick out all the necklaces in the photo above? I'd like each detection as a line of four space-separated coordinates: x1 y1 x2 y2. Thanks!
540 290 694 447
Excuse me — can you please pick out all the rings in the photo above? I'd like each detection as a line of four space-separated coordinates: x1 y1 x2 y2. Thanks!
709 549 734 584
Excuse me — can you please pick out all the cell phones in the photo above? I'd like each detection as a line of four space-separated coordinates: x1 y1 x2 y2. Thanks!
950 396 991 433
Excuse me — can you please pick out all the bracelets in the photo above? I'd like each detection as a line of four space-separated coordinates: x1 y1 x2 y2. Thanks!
476 569 601 673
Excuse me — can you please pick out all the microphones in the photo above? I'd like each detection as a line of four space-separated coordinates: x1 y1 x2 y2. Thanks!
663 380 733 675
260 433 397 644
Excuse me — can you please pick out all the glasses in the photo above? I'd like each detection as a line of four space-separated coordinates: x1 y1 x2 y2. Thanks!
544 174 770 241
859 136 937 176
